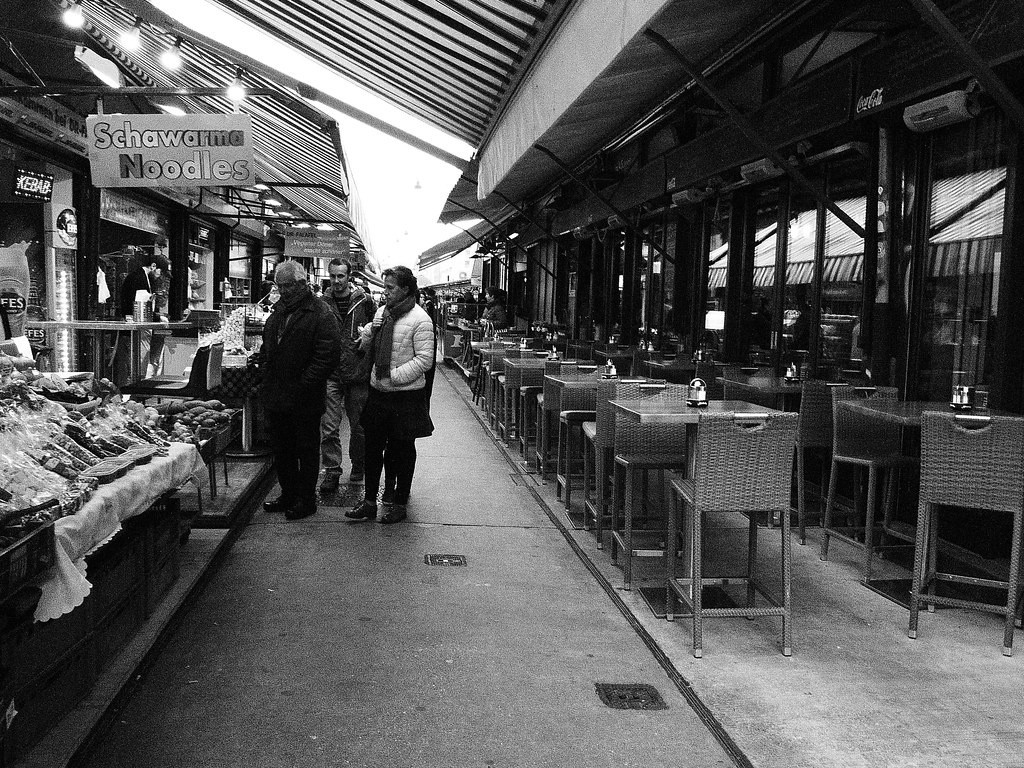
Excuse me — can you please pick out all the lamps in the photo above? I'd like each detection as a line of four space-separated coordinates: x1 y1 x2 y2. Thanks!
575 78 982 241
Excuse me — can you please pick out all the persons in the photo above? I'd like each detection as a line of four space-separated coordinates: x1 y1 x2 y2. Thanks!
258 258 439 522
480 287 507 329
116 255 171 387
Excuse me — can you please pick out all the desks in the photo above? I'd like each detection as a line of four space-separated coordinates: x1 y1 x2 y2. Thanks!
1 439 209 744
717 371 868 408
569 343 615 357
480 348 548 409
500 357 596 458
645 360 728 382
545 375 668 513
470 341 517 370
598 348 677 374
838 394 1009 613
459 325 478 341
609 400 775 618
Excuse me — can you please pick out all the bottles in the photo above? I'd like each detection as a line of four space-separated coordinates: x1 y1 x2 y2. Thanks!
787 368 791 377
690 386 696 399
548 351 556 357
609 365 616 374
606 365 609 373
961 392 969 403
953 391 960 403
697 386 706 400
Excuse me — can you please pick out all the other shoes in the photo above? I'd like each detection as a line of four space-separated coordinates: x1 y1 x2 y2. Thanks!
349 463 365 484
320 473 340 492
285 503 316 519
264 495 295 512
382 492 394 506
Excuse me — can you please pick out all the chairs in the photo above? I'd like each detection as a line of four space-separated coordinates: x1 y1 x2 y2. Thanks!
450 322 1024 657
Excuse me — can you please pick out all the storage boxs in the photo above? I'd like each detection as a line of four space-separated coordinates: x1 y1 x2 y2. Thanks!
196 410 243 463
0 524 55 605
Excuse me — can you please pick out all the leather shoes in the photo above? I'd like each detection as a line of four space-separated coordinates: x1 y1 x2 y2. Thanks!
346 501 377 519
382 507 409 524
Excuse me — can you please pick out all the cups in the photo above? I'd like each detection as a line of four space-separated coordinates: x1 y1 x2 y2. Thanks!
974 391 988 407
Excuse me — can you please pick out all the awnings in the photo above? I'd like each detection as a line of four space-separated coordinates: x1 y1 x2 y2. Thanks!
61 0 565 286
704 165 1006 288
